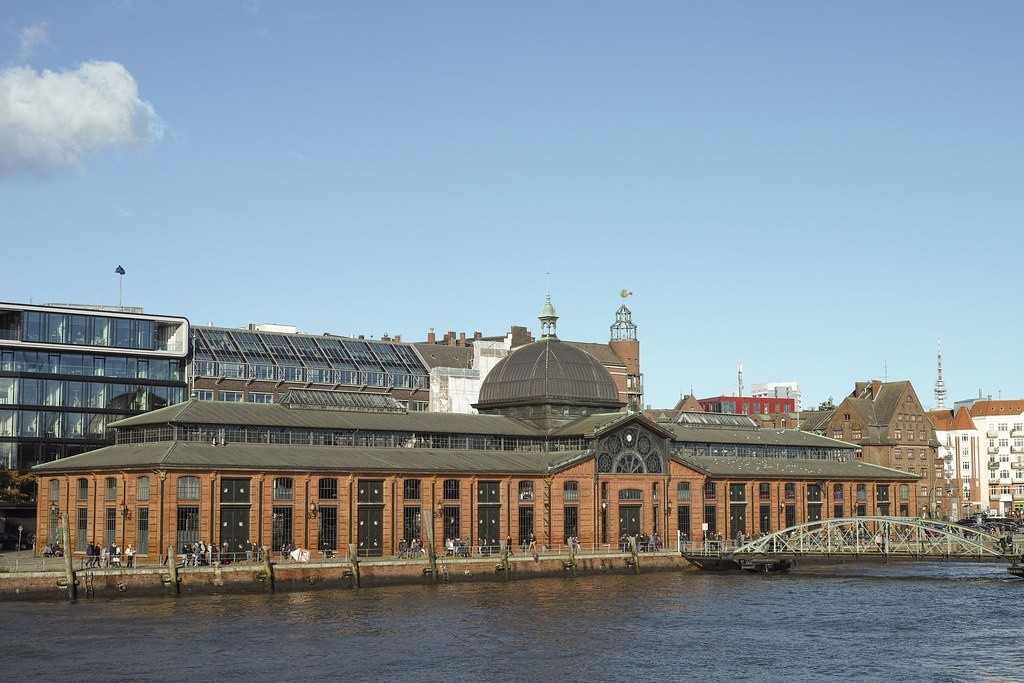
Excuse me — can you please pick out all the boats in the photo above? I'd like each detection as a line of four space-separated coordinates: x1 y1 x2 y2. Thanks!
683 552 792 572
1006 565 1024 578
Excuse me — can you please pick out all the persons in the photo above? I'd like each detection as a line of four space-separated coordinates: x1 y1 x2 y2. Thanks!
40 543 62 558
568 536 582 552
619 532 662 552
397 537 426 560
445 532 539 557
703 512 1024 554
280 543 293 559
322 542 335 559
163 540 264 565
85 541 137 568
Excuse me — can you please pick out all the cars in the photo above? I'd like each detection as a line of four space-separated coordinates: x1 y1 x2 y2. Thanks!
965 522 1018 533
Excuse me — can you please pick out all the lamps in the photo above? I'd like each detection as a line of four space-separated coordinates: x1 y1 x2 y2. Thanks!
601 499 606 515
853 499 859 514
780 499 785 514
307 497 319 519
434 498 444 518
120 500 132 521
49 500 63 519
668 498 672 516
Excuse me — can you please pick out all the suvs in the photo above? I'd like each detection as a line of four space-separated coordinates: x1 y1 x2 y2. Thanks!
956 519 980 525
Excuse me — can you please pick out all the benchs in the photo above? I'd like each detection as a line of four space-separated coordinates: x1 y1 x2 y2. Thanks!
563 545 569 548
600 544 612 548
317 551 339 555
